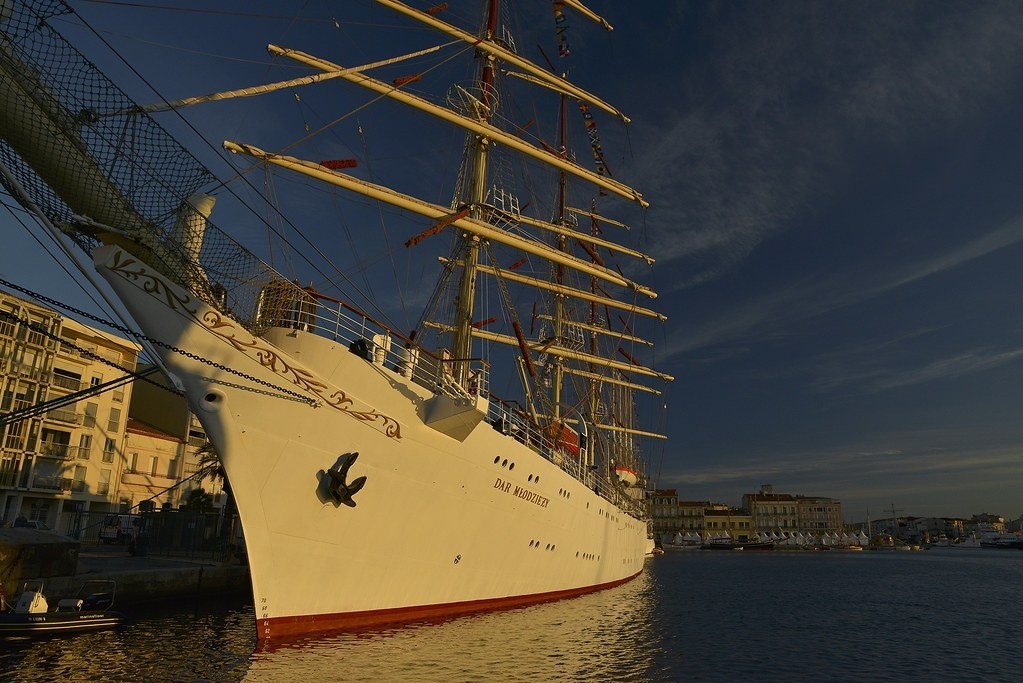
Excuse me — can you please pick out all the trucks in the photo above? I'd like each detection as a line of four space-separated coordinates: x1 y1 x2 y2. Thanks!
99 516 152 545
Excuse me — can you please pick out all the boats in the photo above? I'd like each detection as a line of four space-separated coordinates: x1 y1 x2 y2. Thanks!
710 535 774 551
862 501 1023 551
0 0 674 648
0 579 124 637
